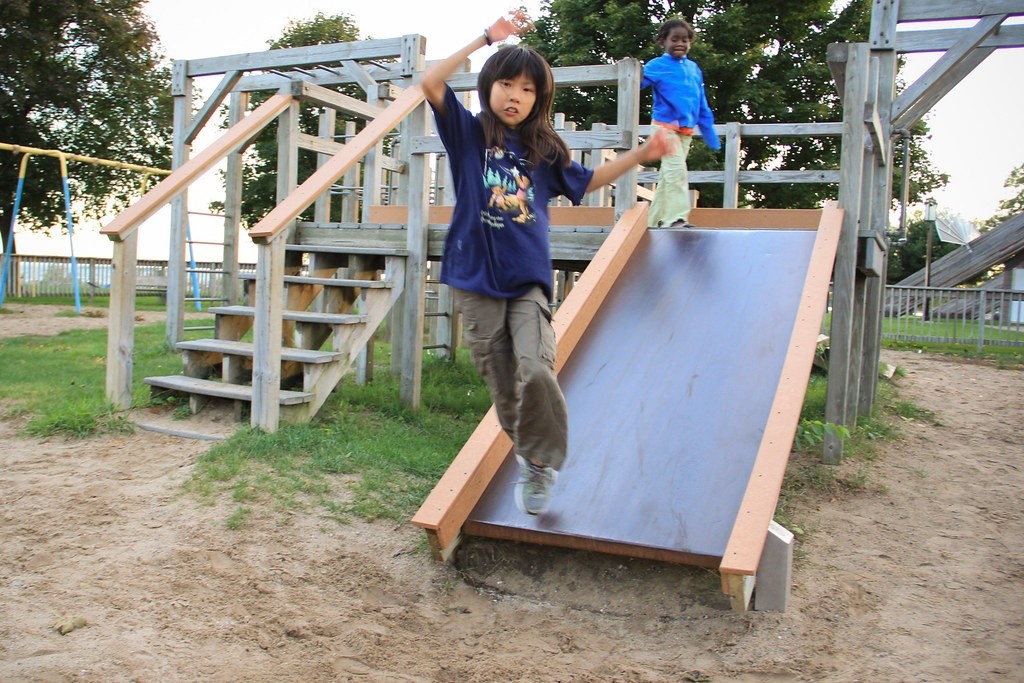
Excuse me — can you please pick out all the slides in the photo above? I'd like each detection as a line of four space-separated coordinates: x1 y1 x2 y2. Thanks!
411 202 846 614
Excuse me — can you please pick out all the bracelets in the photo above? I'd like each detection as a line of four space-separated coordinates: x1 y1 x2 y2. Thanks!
484 28 491 45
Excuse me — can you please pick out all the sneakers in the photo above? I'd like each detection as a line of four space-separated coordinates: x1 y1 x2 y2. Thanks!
511 454 555 515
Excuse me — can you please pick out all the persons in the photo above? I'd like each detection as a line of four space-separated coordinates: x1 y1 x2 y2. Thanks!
613 18 720 228
421 10 674 515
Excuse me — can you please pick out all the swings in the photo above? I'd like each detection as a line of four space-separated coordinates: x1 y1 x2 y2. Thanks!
87 165 120 290
